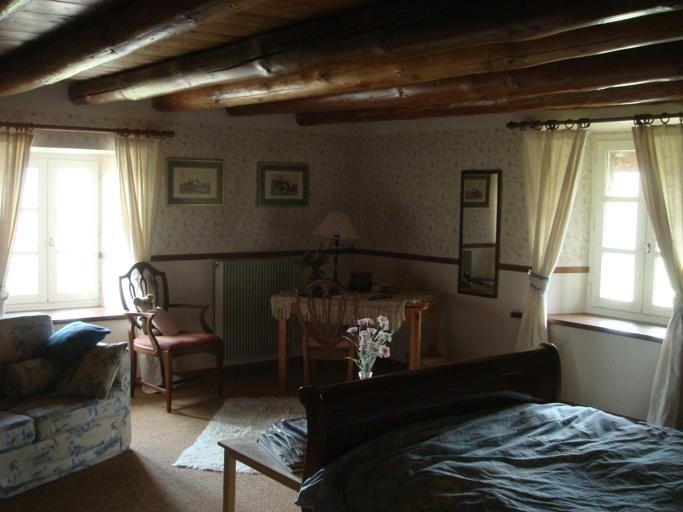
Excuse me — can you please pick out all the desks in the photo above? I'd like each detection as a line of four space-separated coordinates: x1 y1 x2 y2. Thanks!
217 435 303 512
270 288 447 392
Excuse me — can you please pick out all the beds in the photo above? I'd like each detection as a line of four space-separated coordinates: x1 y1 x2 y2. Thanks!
295 343 683 511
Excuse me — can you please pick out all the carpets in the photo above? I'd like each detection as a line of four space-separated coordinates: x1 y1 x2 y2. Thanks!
173 397 308 475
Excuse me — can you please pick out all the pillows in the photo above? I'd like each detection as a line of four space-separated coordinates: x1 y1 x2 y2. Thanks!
0 320 128 407
146 310 192 336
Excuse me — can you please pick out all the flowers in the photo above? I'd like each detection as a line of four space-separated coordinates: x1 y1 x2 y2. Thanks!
341 315 395 378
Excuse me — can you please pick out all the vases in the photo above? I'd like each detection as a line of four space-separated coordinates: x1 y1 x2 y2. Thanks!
357 371 373 381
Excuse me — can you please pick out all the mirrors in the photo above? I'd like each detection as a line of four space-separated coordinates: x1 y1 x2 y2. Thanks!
457 169 501 298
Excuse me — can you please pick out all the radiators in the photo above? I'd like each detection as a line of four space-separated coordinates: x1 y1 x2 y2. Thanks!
214 256 296 366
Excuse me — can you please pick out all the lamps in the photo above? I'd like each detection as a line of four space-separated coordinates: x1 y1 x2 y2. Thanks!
313 212 359 295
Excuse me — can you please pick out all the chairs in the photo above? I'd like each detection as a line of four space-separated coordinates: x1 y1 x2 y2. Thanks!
118 263 224 413
293 278 359 387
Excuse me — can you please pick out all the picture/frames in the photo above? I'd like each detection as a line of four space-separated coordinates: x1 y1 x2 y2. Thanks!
463 175 489 208
162 158 229 208
258 161 309 206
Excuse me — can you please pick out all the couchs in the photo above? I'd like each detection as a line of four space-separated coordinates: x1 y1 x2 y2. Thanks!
0 311 132 498
